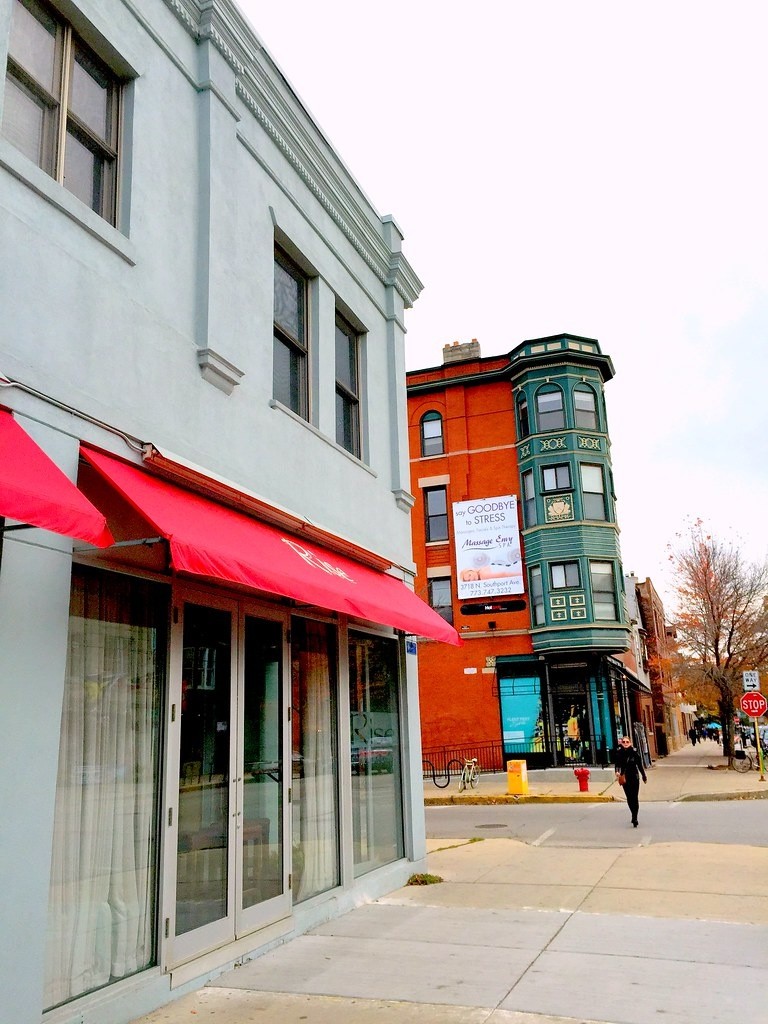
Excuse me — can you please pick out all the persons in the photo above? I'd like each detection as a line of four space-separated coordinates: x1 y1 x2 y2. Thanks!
687 723 750 748
614 736 648 827
566 713 582 759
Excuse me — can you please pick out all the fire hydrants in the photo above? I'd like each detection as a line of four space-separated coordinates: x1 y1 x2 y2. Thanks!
574 766 591 792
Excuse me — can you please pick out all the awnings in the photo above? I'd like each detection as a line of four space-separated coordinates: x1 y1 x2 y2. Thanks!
77 444 467 647
0 408 116 550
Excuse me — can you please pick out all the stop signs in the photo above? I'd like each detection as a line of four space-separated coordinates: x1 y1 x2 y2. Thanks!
740 691 768 718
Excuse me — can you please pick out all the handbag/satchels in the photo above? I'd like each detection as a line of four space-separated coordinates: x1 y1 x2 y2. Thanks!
618 773 626 785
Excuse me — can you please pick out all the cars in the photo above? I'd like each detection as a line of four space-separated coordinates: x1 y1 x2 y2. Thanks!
740 724 768 749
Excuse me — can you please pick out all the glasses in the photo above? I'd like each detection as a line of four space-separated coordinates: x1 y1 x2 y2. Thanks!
622 741 629 743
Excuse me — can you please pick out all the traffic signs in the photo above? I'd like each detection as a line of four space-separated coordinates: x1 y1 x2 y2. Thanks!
743 670 759 691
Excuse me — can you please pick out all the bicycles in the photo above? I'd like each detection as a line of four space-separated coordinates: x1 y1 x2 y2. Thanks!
732 749 768 774
457 757 481 793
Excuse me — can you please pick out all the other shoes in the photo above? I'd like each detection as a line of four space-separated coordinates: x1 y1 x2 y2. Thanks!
631 816 638 828
